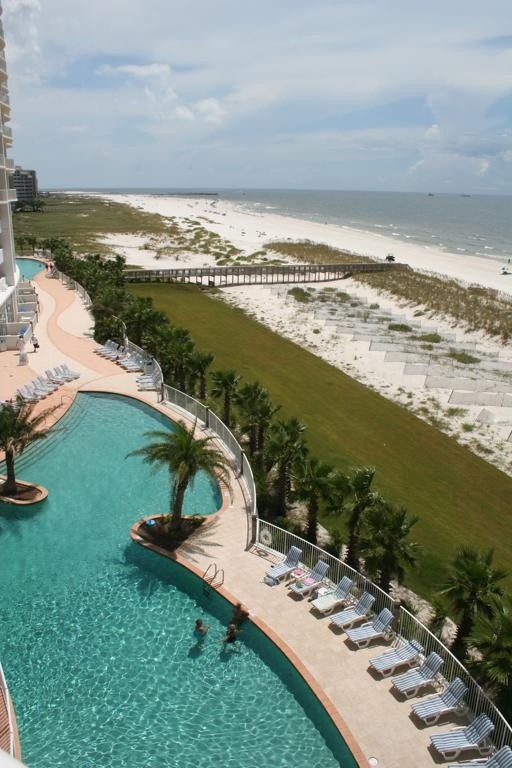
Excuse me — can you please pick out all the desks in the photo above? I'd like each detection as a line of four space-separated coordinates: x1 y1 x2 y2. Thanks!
316 585 331 597
290 566 306 578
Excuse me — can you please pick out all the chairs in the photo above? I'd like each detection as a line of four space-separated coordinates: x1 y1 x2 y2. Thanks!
15 363 81 404
346 606 394 649
447 744 512 767
312 574 353 616
411 677 470 726
331 588 376 633
391 651 444 700
427 711 497 759
287 559 329 601
368 637 426 679
263 543 303 583
93 338 162 393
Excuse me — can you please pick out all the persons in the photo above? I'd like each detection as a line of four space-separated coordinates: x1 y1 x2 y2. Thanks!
232 603 249 623
224 623 242 643
195 619 211 636
15 335 26 352
30 333 39 352
